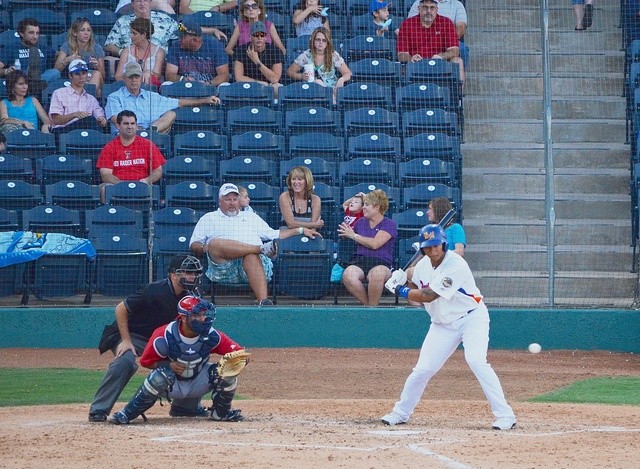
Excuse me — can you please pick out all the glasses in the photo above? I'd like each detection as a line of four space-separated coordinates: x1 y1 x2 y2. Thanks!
314 38 327 42
251 33 266 38
419 5 439 10
243 3 260 9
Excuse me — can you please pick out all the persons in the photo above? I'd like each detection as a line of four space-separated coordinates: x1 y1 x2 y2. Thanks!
96 110 167 204
338 189 397 307
0 71 51 138
1 16 51 84
329 192 364 284
50 60 107 134
287 27 352 105
408 197 466 288
406 0 468 82
103 0 181 72
189 184 322 308
88 254 203 421
291 1 331 39
366 1 399 39
114 1 176 15
178 0 237 16
280 165 322 240
381 222 516 431
232 20 287 96
104 61 220 135
235 185 255 213
395 0 460 65
114 17 164 84
55 17 106 99
566 3 596 31
225 1 285 61
110 296 246 423
165 18 229 87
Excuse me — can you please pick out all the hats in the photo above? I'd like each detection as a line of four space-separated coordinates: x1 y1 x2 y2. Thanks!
173 20 202 37
219 183 240 196
369 0 391 14
122 61 142 77
68 58 88 73
249 20 266 34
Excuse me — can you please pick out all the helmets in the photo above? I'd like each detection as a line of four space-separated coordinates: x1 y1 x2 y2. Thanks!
169 254 204 292
176 295 216 337
418 223 447 255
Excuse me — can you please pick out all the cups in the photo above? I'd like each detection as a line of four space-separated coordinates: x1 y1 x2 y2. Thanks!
304 65 314 84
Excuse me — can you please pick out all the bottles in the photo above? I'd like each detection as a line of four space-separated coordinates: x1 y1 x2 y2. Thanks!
13 58 21 69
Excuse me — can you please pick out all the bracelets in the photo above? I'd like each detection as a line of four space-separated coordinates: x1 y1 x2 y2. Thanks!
298 226 304 235
397 286 410 300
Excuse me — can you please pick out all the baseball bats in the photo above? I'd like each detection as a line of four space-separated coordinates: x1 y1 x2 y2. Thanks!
403 208 457 273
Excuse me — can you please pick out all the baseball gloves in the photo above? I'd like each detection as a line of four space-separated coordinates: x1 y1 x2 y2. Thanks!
216 348 250 379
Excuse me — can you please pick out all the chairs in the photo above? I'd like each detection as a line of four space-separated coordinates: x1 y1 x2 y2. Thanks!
0 0 462 305
620 0 640 274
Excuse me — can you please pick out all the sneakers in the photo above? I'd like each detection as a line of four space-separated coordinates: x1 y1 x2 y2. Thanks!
89 413 107 422
381 409 406 424
210 409 244 422
110 411 128 424
262 238 278 257
491 417 516 430
258 298 273 305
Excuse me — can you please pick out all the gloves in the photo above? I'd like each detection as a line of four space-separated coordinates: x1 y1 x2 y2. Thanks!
384 278 402 295
392 267 408 285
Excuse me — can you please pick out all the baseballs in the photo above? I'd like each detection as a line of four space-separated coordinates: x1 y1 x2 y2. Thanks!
527 342 542 354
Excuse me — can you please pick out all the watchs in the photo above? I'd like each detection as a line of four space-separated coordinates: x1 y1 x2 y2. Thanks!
256 61 262 68
